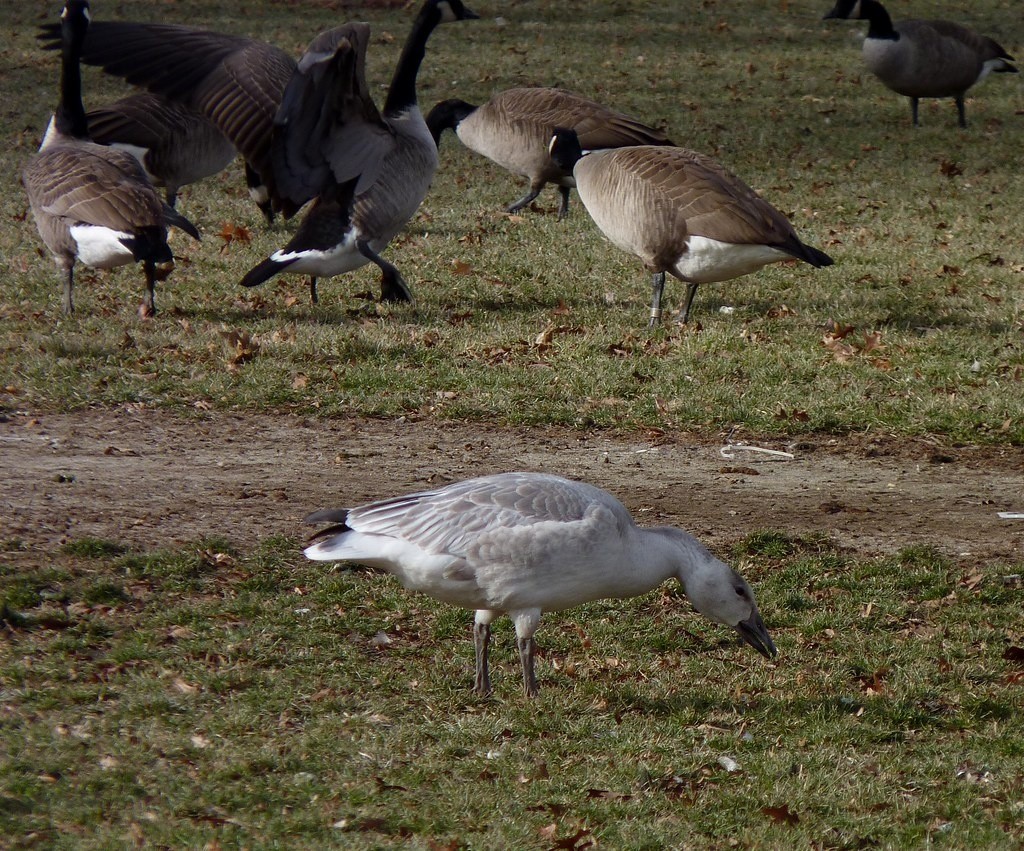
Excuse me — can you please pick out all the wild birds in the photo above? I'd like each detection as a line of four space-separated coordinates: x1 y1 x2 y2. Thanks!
86 92 240 208
23 0 203 319
425 88 676 222
821 0 1021 128
302 470 778 698
548 126 835 329
34 0 481 304
